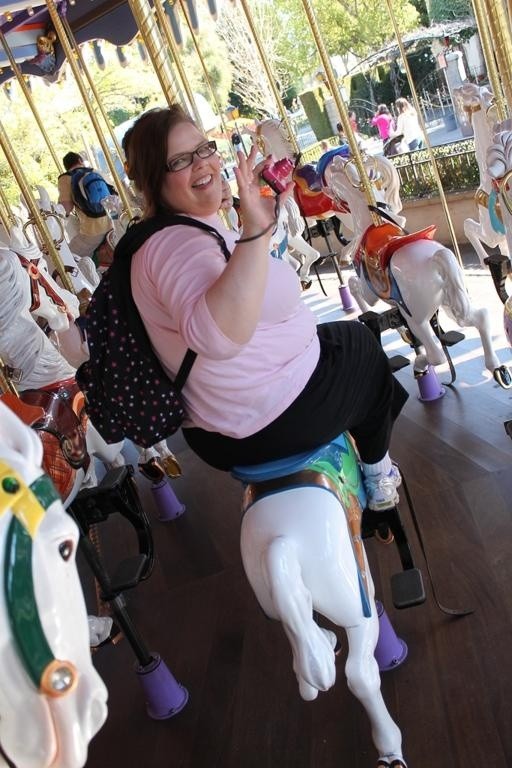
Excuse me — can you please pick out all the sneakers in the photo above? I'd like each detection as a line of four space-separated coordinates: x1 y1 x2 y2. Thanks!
363 457 403 513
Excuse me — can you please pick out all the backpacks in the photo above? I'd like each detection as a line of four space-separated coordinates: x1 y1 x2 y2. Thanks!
74 213 233 451
58 167 119 219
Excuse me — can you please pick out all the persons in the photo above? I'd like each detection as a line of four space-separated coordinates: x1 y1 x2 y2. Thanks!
57 150 115 260
124 108 411 515
336 122 349 146
348 111 357 134
395 97 424 151
372 104 396 146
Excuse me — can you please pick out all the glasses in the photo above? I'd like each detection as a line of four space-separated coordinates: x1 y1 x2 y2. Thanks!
164 140 218 173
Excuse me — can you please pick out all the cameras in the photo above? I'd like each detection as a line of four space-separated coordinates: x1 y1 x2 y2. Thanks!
260 156 295 194
